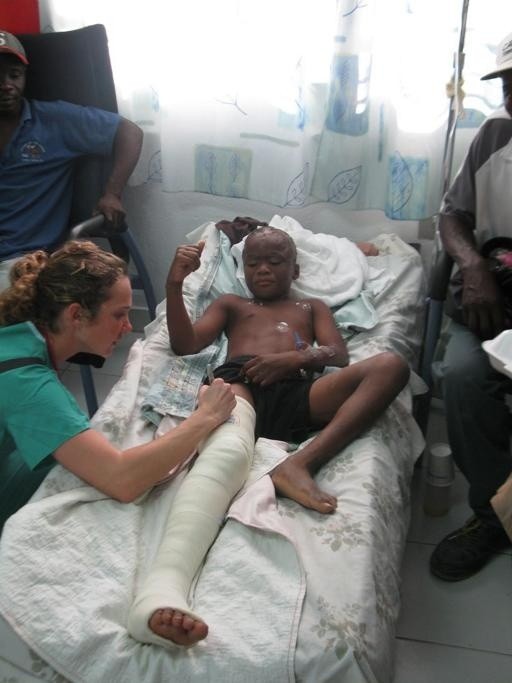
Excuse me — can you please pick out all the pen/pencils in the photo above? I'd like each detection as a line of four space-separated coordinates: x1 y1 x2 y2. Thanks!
207 364 215 384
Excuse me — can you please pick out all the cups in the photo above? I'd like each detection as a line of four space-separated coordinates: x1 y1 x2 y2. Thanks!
420 479 455 518
425 441 456 488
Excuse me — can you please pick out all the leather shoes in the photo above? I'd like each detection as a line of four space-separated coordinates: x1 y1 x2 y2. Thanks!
430 516 512 581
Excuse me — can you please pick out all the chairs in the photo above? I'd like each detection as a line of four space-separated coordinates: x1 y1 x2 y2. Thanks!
12 23 157 421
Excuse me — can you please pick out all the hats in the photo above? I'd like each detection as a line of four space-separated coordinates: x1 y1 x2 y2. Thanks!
0 29 29 65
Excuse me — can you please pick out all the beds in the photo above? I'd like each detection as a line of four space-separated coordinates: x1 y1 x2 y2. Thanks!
0 221 424 683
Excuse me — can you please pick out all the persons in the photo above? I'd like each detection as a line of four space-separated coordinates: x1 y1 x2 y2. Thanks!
429 33 512 584
0 240 238 528
124 224 411 653
1 29 144 295
